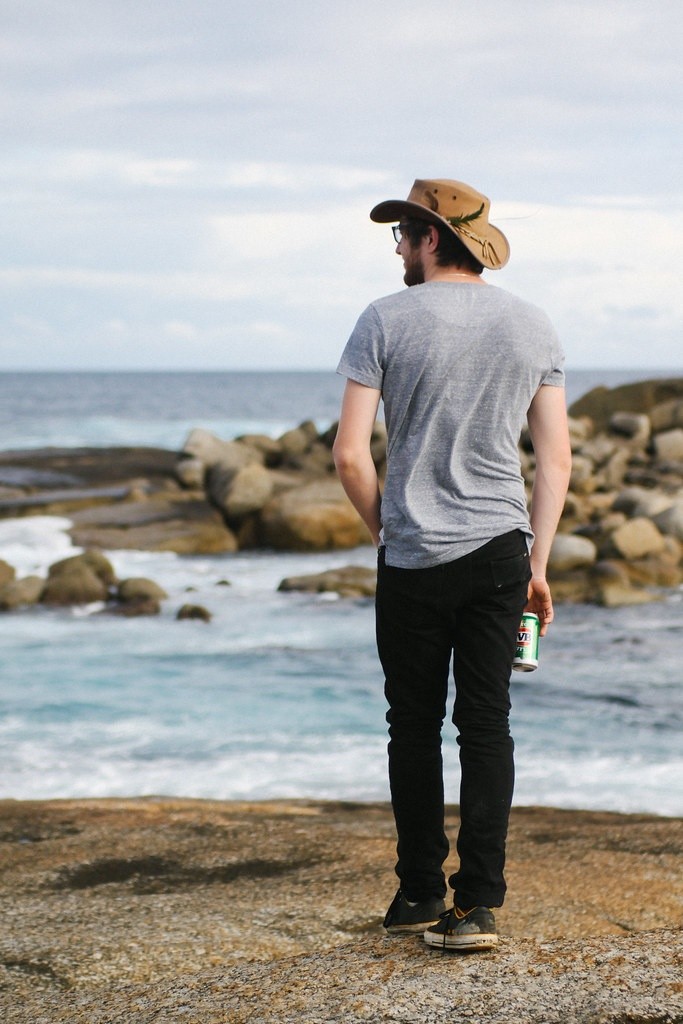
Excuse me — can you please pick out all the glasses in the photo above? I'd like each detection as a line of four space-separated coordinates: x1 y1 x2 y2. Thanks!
392 225 426 243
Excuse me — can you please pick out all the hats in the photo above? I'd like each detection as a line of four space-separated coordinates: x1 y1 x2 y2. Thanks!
370 179 511 270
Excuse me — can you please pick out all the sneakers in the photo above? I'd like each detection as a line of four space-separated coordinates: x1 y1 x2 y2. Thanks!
423 905 498 955
383 888 446 933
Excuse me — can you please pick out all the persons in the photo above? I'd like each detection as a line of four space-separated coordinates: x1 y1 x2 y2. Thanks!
332 180 573 950
